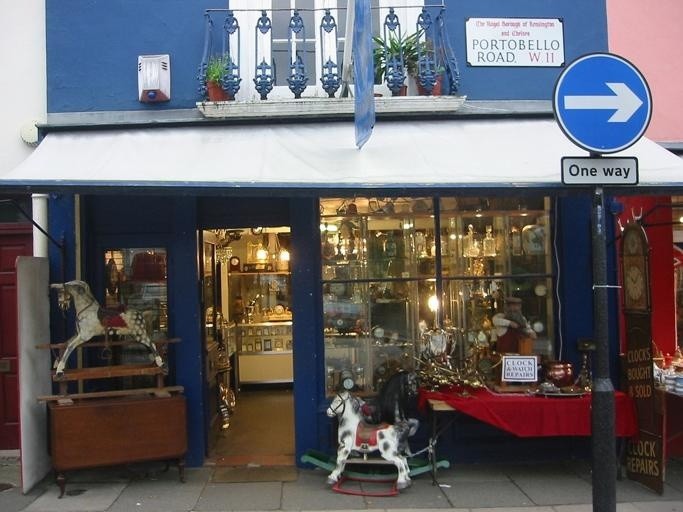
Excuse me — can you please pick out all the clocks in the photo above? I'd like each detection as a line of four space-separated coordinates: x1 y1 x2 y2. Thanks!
621 255 647 311
230 256 241 272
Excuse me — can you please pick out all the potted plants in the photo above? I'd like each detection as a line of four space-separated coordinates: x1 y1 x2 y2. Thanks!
416 66 446 97
371 28 433 97
205 54 234 101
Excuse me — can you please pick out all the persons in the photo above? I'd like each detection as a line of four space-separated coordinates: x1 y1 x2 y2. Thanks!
492 296 539 355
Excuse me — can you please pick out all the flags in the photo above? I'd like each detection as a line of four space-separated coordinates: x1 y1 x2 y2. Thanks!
349 0 375 150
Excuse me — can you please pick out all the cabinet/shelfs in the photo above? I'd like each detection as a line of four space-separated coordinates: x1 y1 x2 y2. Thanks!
237 323 293 391
319 192 555 398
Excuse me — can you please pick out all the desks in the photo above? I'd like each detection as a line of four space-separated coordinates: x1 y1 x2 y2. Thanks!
425 389 628 487
47 394 187 499
